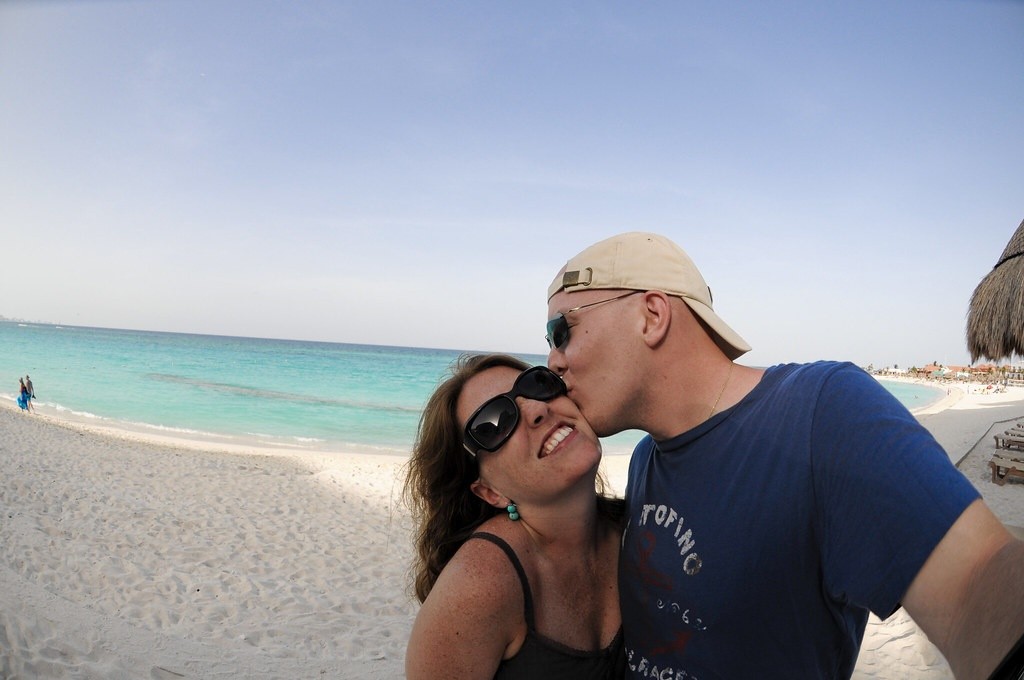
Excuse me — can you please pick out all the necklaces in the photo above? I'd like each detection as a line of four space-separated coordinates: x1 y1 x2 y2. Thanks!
708 361 736 419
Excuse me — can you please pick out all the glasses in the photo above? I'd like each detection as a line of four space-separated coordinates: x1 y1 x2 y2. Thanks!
463 365 567 462
545 288 650 349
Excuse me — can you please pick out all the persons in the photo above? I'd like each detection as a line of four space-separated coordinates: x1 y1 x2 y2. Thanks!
405 354 626 680
17 375 35 414
547 232 1024 680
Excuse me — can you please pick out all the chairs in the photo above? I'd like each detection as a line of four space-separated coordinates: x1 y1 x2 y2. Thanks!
988 421 1024 486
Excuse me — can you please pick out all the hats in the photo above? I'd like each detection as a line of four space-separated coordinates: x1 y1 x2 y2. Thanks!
547 231 751 362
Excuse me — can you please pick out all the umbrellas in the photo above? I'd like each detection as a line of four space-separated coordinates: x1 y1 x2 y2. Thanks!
964 219 1024 366
